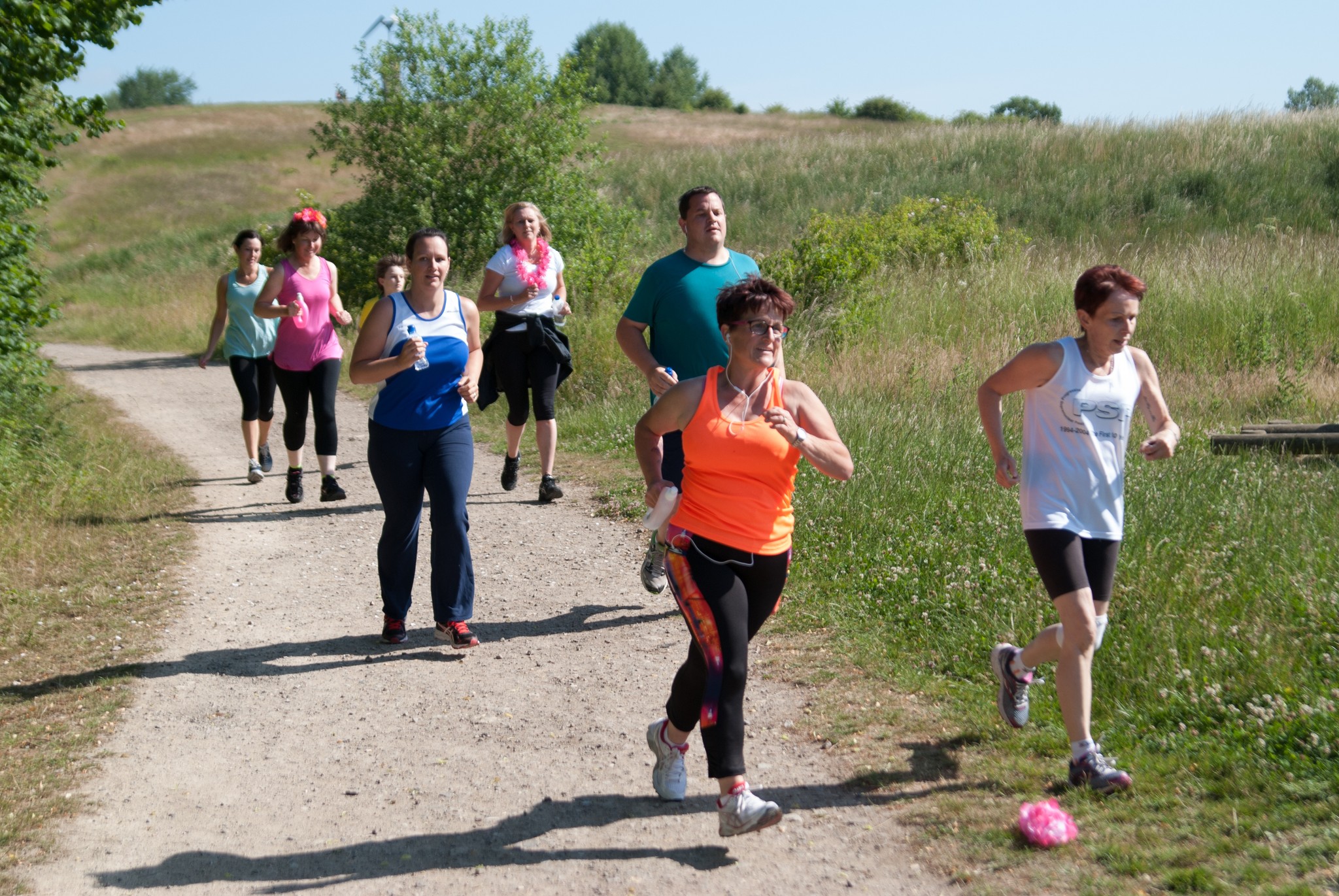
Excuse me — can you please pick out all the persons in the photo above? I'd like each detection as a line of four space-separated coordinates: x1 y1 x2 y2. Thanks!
199 229 282 483
253 208 352 504
633 275 854 838
476 201 571 503
617 186 760 595
349 227 482 650
976 265 1178 796
357 255 409 336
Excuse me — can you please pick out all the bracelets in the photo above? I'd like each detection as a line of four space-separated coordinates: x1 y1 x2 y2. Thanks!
510 295 516 306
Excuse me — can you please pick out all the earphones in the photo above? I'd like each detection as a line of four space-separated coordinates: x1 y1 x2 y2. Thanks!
726 333 730 344
685 225 687 232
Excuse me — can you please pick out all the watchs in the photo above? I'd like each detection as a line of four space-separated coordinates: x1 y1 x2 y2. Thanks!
791 427 805 447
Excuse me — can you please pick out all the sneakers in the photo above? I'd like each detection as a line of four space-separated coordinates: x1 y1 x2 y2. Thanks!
285 464 304 503
538 474 563 501
321 474 346 502
501 449 522 490
1068 753 1133 789
647 719 689 800
248 459 264 483
380 612 407 642
435 617 479 650
258 442 273 473
716 783 783 835
992 644 1034 730
640 529 669 594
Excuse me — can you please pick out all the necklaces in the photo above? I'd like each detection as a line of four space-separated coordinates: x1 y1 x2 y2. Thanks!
510 237 550 289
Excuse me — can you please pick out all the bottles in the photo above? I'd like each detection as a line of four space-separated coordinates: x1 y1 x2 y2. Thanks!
654 367 673 406
407 325 429 371
642 487 678 530
292 292 309 328
552 295 566 326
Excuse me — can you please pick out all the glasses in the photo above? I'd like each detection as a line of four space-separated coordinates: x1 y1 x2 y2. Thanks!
729 320 789 340
